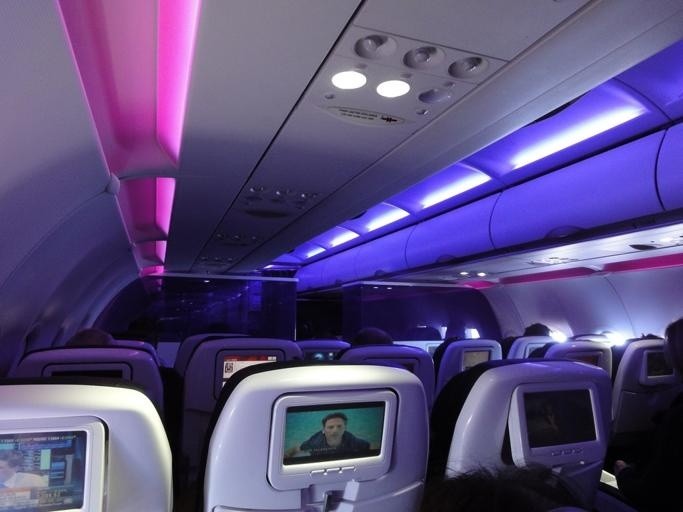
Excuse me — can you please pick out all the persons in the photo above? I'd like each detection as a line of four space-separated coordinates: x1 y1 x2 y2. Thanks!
417 461 590 511
335 302 549 374
285 412 372 460
612 315 682 512
61 305 233 436
528 396 592 442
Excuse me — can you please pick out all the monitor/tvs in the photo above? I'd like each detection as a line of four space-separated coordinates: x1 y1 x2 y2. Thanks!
462 346 496 371
43 363 131 383
268 390 397 491
508 382 607 469
639 349 679 386
214 348 285 399
0 417 105 512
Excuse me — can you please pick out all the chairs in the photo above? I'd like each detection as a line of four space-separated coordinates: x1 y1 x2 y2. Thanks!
0 334 681 511
